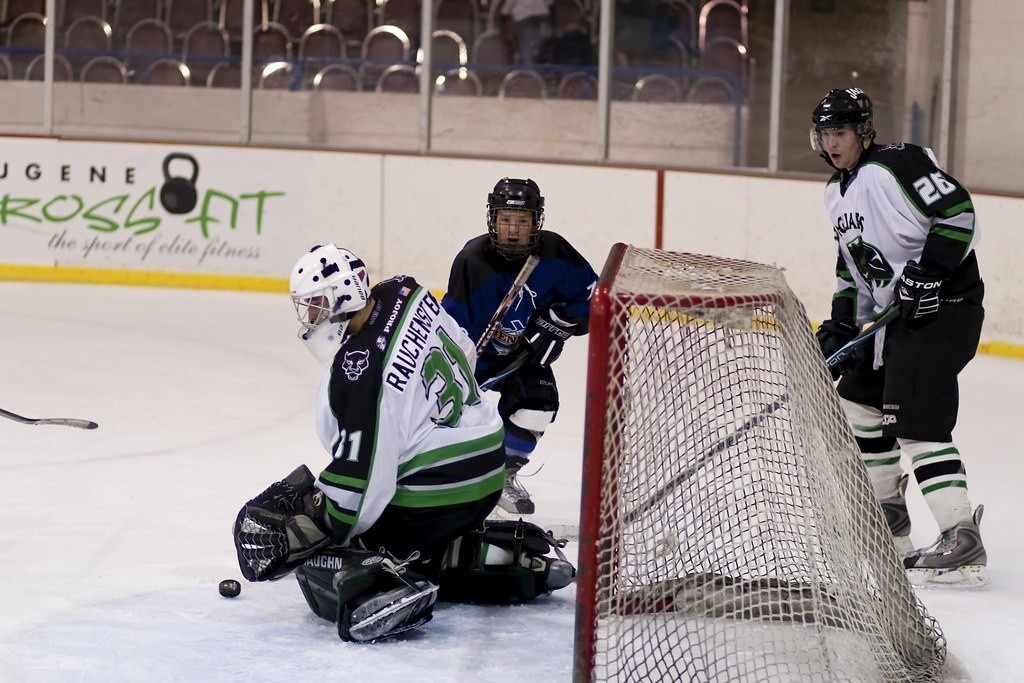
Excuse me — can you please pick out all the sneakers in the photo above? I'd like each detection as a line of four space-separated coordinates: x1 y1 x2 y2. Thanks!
903 504 992 591
496 454 535 519
881 474 915 557
531 556 577 591
349 581 440 640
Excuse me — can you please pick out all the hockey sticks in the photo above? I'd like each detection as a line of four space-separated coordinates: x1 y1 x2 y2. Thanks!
478 350 530 392
473 255 541 360
0 409 99 430
542 304 902 544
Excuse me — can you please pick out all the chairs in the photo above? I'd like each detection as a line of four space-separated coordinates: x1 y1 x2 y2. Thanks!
0 0 757 106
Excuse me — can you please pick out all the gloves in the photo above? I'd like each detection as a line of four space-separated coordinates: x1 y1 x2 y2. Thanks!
816 320 860 381
525 303 578 369
894 260 942 318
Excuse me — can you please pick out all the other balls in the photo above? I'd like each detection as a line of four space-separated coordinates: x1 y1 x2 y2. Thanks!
218 579 241 598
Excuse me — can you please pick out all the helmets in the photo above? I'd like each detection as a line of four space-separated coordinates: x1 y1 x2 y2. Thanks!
290 243 370 363
812 87 874 138
488 177 545 260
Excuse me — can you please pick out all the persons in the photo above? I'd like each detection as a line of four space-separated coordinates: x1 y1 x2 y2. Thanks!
442 177 599 513
488 0 599 66
233 244 575 644
810 85 989 589
